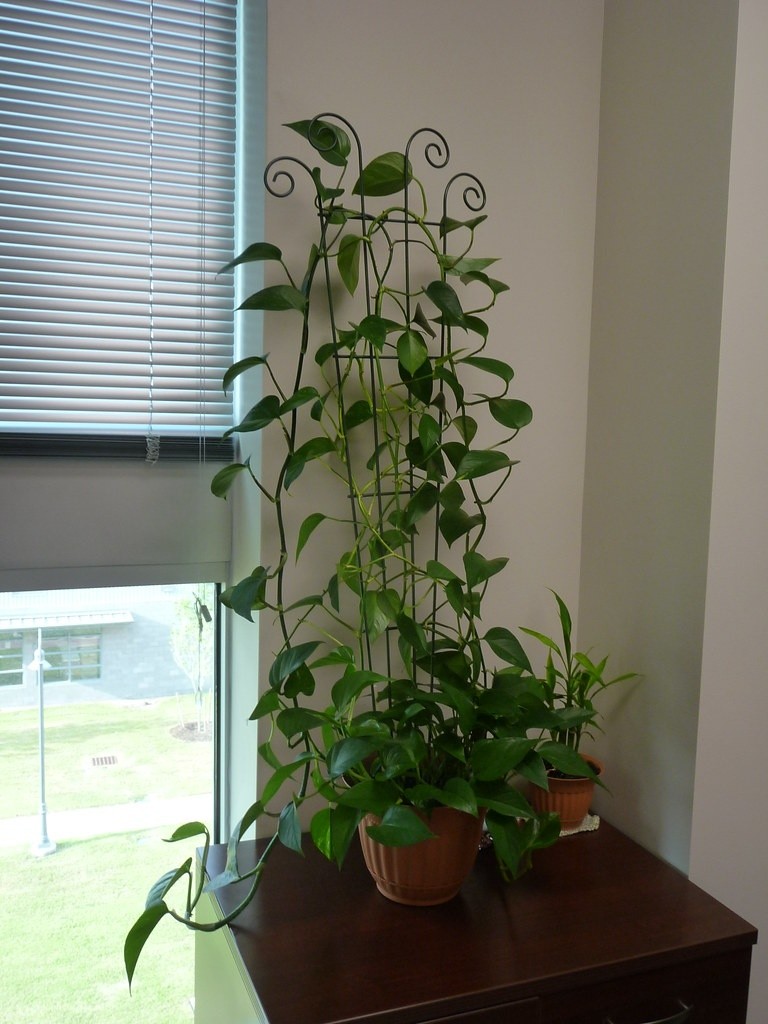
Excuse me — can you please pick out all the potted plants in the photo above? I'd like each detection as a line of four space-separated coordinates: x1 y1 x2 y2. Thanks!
122 117 612 996
518 588 647 830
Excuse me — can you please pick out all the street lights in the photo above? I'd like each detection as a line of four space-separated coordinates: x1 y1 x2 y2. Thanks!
29 648 57 855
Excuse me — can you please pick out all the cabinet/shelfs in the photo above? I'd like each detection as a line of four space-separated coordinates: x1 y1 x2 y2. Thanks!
194 828 758 1024
